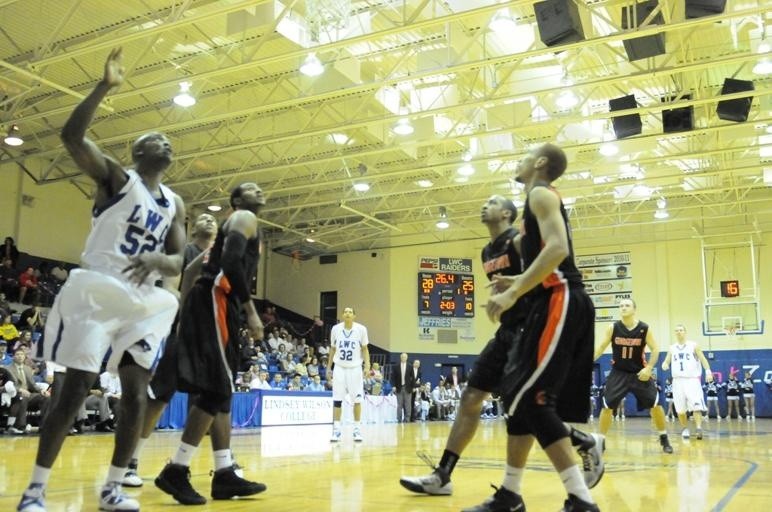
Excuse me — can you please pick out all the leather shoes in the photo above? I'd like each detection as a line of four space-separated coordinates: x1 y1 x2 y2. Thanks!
103 424 115 432
78 423 85 433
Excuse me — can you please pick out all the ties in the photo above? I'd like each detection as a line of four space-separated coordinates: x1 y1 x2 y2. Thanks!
19 368 25 389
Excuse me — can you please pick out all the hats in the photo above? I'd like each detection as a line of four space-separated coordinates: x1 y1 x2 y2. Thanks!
0 340 7 345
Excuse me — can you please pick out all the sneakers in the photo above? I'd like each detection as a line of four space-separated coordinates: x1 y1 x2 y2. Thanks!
738 416 742 419
353 432 362 441
696 432 702 439
559 493 601 512
690 416 694 421
660 434 673 453
665 416 669 421
746 416 750 419
120 468 143 487
752 416 755 419
211 460 266 499
444 414 456 421
682 428 690 438
331 431 341 441
69 427 78 435
155 464 206 505
717 416 722 419
400 450 453 495
725 416 731 419
616 416 619 418
461 484 526 512
0 426 30 434
480 413 501 419
611 416 614 418
98 481 140 512
577 433 605 489
672 416 675 421
590 415 593 418
622 416 625 418
704 415 709 420
18 489 47 512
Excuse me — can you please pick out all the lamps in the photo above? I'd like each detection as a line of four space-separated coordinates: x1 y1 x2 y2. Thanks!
435 207 450 228
353 164 369 191
174 83 196 107
3 124 24 146
394 117 413 134
654 200 669 218
300 54 322 76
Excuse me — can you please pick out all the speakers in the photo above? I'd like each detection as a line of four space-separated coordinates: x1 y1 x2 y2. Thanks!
534 0 585 54
684 1 727 19
610 94 642 139
621 0 666 61
661 94 695 134
716 77 755 123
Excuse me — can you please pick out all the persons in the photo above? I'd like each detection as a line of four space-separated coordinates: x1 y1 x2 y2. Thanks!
362 361 396 397
594 298 674 454
233 307 334 391
6 348 50 434
616 398 625 419
0 312 20 353
0 256 18 302
0 292 17 322
410 360 467 422
464 143 601 512
0 340 13 366
34 261 50 283
662 325 713 441
75 372 115 434
589 379 599 417
15 301 46 334
326 306 370 442
11 330 34 359
482 394 495 419
664 378 676 422
52 262 68 288
741 372 755 420
98 367 124 427
152 182 268 506
121 212 247 488
0 236 20 272
399 196 609 493
18 342 44 385
14 46 187 512
721 374 743 421
702 376 722 420
0 366 24 435
392 352 416 423
18 266 38 306
